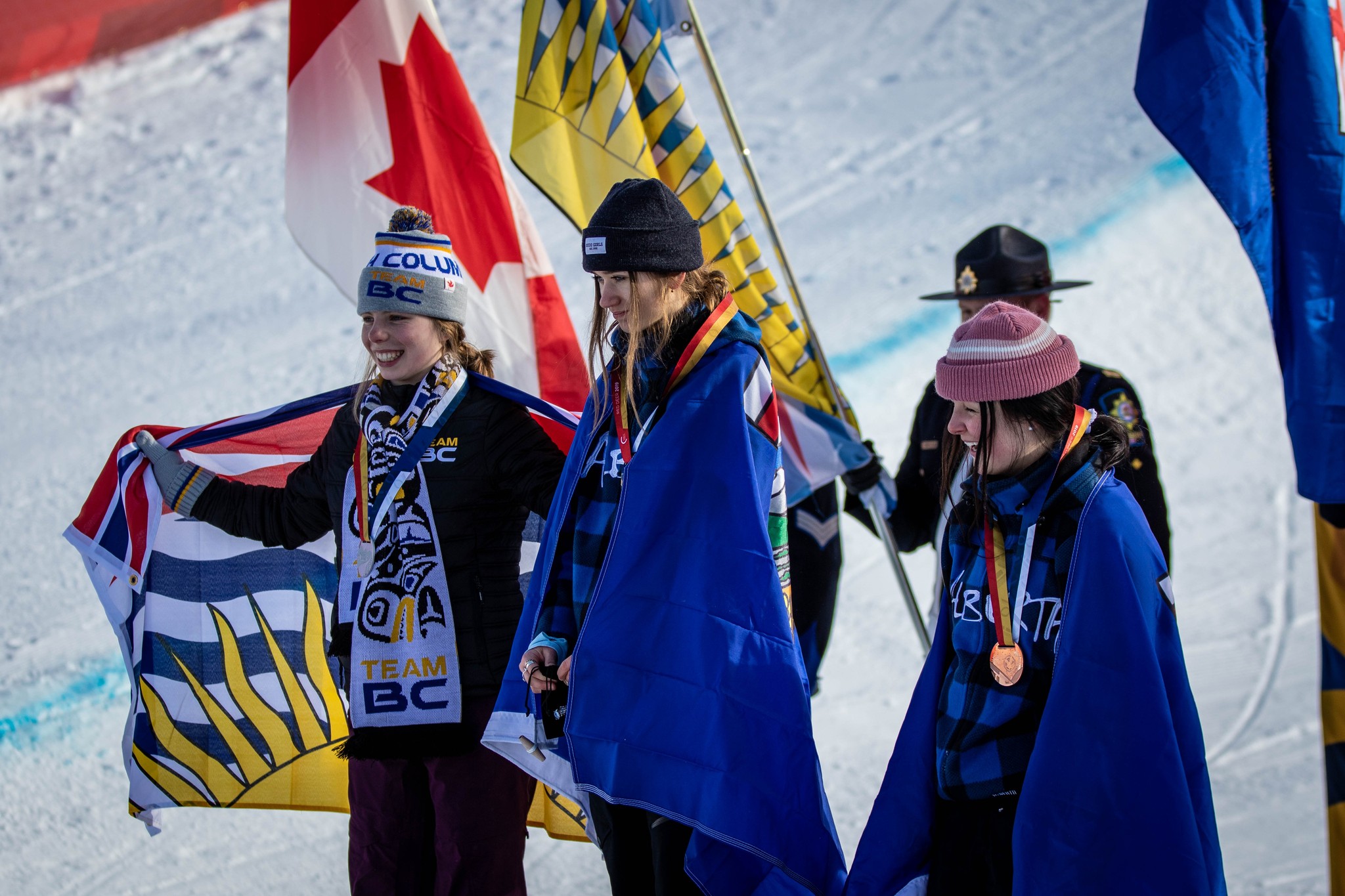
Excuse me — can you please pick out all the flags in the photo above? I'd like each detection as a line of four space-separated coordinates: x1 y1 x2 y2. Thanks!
509 0 866 696
1133 0 1344 505
285 0 589 416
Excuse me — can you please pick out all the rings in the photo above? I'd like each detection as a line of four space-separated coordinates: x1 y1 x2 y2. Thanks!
564 680 569 686
524 658 536 672
522 671 526 682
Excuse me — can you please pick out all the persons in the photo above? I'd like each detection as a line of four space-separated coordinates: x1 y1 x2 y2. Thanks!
136 205 581 896
520 179 841 895
844 226 1172 626
837 299 1229 896
787 476 848 694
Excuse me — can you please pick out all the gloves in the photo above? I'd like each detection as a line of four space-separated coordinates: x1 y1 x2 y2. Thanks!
840 440 884 496
135 429 219 520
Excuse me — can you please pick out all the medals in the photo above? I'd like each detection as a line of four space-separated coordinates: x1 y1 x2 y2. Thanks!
356 536 375 577
990 640 1023 687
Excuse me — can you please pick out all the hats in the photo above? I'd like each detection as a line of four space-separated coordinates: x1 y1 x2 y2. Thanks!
936 300 1083 402
922 225 1090 300
584 178 705 275
358 206 468 326
